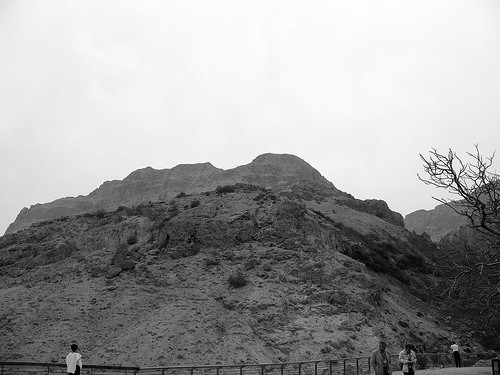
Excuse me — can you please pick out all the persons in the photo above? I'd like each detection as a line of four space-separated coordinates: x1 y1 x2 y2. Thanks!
450 341 461 368
372 340 393 375
66 344 82 375
398 344 417 375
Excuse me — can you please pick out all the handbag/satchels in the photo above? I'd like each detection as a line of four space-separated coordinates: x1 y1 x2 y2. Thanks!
74 365 80 375
